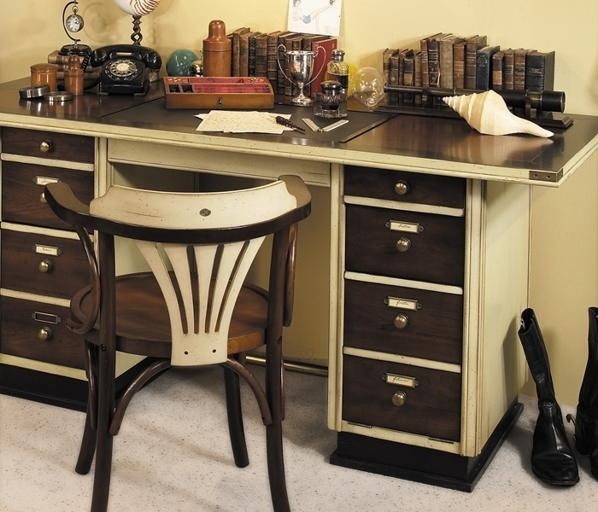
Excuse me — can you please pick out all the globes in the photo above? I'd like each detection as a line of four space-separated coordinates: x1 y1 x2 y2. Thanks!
165 48 200 76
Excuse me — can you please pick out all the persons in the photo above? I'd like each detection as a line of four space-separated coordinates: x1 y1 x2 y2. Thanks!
307 0 340 34
290 0 308 30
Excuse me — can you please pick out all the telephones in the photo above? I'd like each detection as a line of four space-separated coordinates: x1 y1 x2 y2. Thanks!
89 43 162 96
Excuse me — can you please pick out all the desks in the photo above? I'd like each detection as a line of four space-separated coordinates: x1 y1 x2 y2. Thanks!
0 77 598 494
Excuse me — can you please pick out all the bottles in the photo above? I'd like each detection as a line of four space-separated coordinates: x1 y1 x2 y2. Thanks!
204 20 231 77
324 50 349 114
65 57 84 96
319 81 342 117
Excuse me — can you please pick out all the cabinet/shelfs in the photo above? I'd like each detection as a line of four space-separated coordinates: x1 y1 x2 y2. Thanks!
1 128 202 414
330 163 472 457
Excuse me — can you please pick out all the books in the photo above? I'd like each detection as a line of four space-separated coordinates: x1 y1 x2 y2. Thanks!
226 26 338 105
382 32 556 120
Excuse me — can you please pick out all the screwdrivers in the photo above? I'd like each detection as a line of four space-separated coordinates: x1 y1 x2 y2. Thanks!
276 116 306 132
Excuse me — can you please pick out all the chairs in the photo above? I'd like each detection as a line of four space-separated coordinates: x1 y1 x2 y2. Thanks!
43 175 310 512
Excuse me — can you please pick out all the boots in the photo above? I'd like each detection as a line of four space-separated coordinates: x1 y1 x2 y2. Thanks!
518 308 579 486
576 306 597 477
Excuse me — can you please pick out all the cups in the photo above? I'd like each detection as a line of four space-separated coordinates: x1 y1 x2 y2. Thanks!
30 64 58 94
